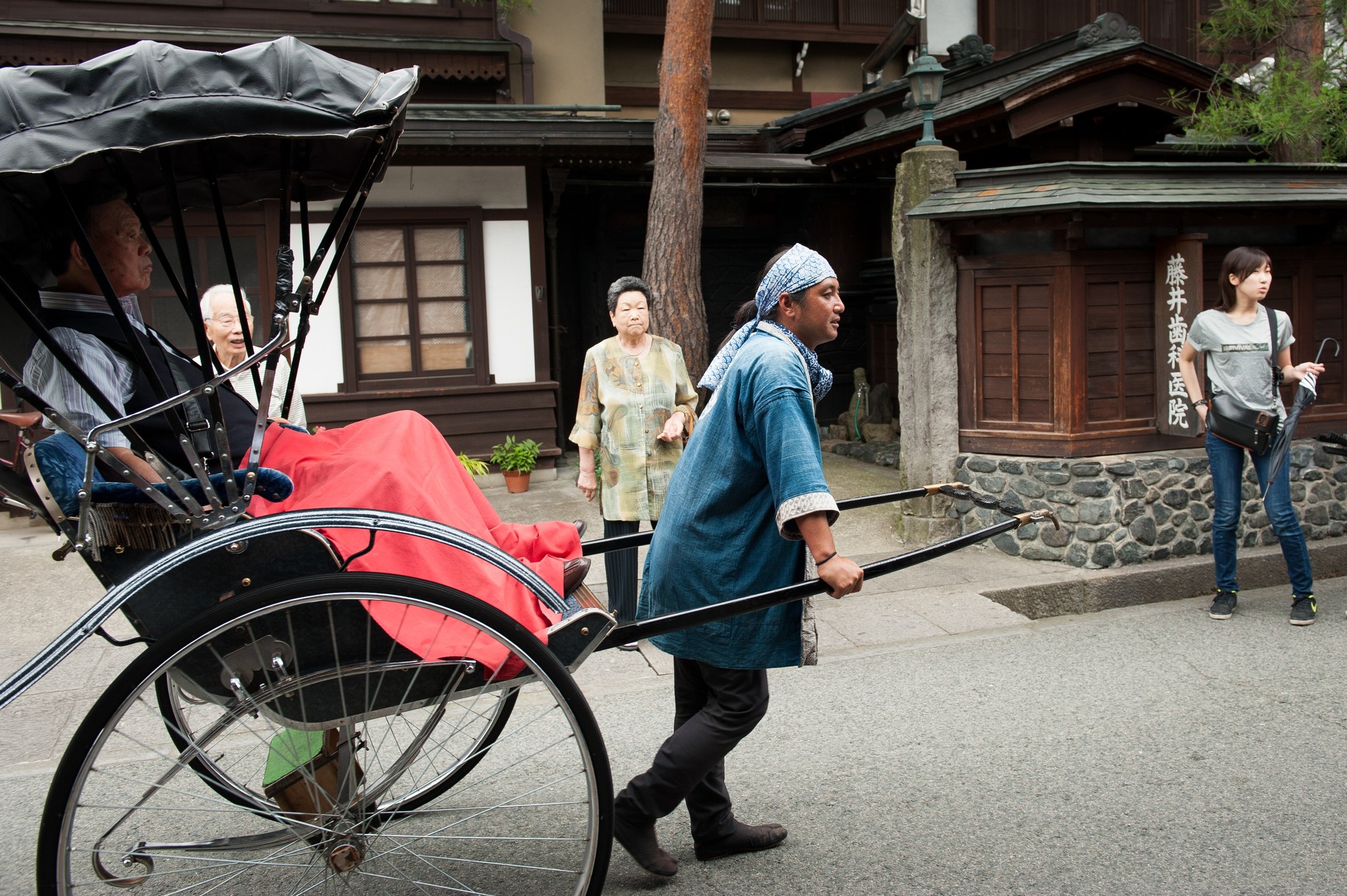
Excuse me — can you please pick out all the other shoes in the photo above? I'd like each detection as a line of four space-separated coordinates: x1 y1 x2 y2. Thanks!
615 816 679 875
694 823 788 861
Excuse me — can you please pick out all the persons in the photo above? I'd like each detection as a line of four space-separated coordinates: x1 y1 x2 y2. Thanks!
569 272 702 651
186 280 311 430
606 244 869 880
1179 246 1329 625
24 188 595 665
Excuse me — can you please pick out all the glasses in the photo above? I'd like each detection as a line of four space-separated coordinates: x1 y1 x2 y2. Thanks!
204 314 254 327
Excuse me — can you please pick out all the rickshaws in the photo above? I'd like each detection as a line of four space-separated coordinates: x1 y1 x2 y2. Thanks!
0 40 1056 896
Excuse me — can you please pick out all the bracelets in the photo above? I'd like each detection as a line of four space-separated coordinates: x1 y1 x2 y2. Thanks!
1190 399 1208 410
816 552 838 566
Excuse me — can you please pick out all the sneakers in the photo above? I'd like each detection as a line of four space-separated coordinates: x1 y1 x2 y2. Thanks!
1210 588 1238 619
1289 594 1317 625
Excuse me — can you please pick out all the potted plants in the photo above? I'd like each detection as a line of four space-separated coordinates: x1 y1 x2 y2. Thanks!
489 434 544 493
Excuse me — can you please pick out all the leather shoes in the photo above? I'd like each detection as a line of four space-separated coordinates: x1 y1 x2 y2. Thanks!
563 557 591 600
572 519 587 539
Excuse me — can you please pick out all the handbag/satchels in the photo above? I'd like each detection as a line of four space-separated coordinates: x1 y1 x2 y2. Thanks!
1205 393 1280 455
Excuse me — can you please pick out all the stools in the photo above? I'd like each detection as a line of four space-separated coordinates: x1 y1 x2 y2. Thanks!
261 725 367 829
